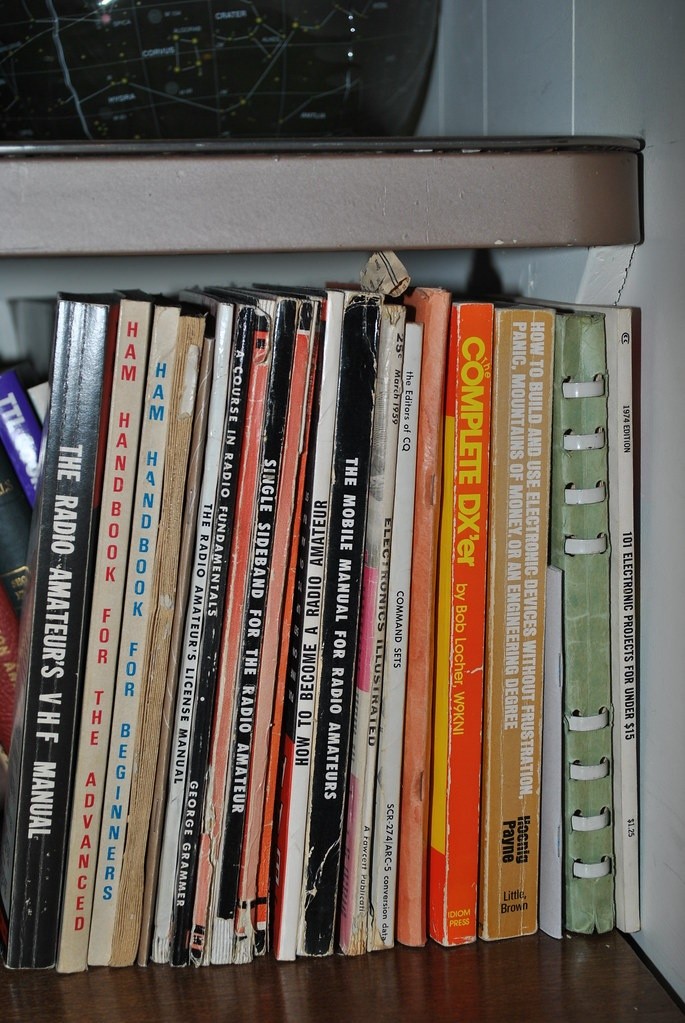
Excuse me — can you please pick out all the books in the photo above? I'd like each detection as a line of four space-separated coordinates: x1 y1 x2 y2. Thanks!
0 278 641 975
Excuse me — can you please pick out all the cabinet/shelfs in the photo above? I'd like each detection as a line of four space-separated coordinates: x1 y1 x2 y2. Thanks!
0 1 685 1023
